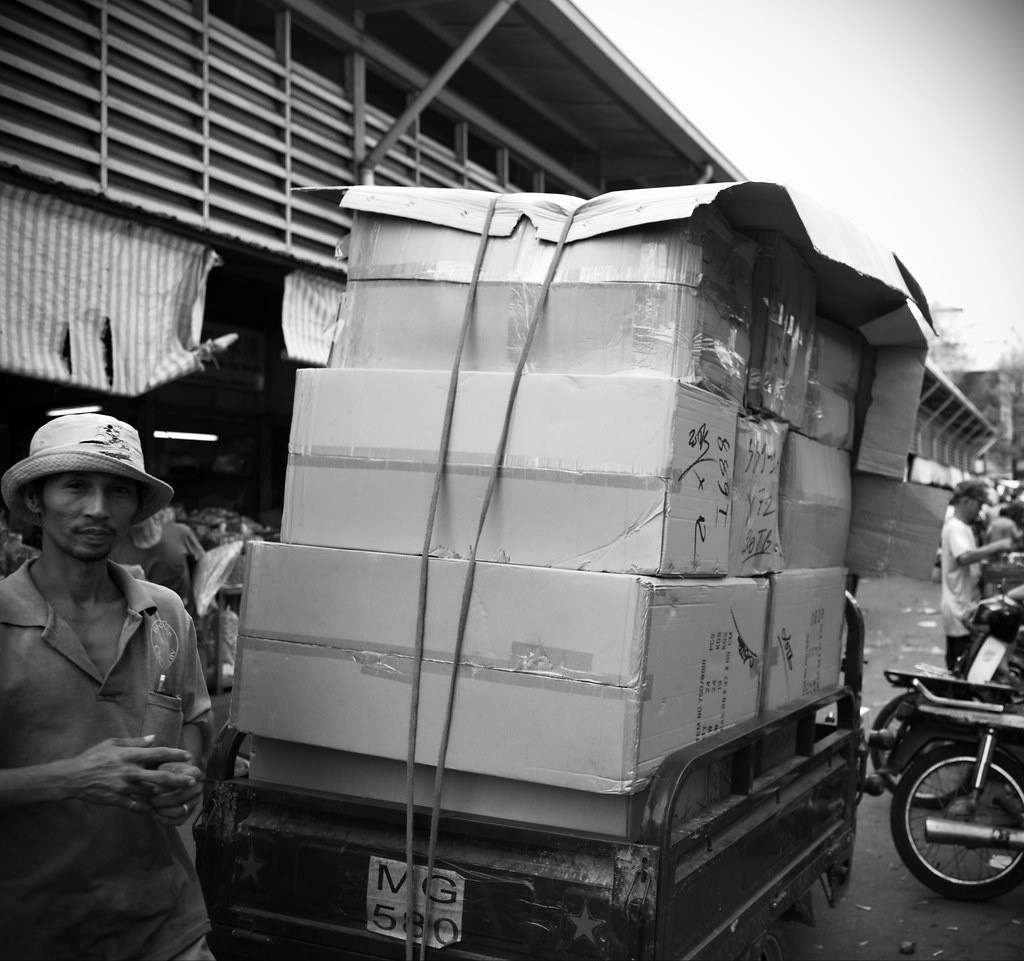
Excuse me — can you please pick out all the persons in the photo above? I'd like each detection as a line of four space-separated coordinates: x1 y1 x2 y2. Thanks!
105 509 207 608
0 413 216 960
940 471 1024 678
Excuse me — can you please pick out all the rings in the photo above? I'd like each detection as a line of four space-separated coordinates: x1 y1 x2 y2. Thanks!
181 803 190 815
129 801 136 808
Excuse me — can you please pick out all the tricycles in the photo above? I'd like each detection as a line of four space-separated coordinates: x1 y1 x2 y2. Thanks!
197 590 883 961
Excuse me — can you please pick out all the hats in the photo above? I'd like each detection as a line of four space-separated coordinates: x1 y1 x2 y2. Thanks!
1 414 174 528
954 481 993 508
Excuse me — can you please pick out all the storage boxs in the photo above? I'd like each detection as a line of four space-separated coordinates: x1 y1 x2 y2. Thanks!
233 200 861 844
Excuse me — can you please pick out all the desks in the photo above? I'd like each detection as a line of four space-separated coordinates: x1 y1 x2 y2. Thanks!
214 588 243 696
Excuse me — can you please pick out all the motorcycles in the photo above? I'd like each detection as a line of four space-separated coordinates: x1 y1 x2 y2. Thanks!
861 582 1024 904
